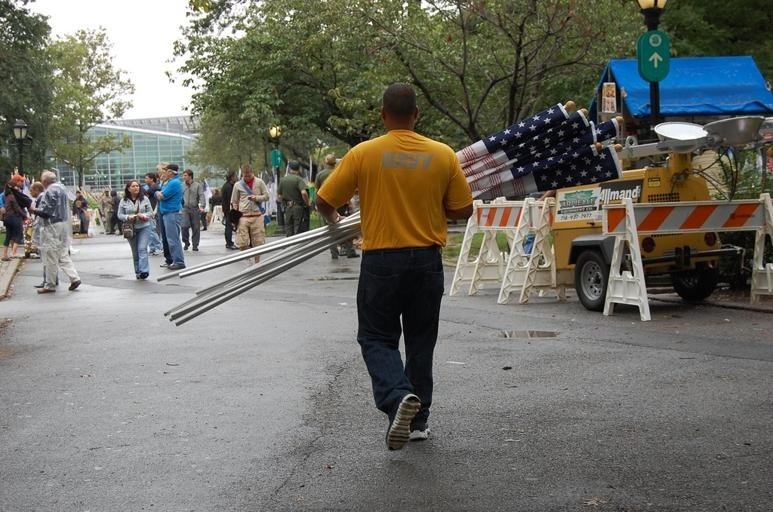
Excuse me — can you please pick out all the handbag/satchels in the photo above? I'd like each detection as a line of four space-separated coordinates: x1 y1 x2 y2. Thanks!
264 216 271 225
121 222 137 239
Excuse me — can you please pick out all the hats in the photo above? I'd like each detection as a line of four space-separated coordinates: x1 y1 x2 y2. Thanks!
162 162 180 173
289 159 300 172
12 173 27 183
325 154 336 165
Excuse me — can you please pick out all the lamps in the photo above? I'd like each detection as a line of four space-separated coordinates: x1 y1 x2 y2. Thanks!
701 114 765 149
653 115 707 152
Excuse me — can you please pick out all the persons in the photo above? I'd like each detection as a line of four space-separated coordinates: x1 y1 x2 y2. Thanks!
2 170 80 294
75 191 89 234
316 82 474 451
316 154 359 259
277 159 309 237
103 161 206 280
206 163 269 263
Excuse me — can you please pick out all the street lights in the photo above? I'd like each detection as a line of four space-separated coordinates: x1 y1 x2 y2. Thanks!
266 124 283 188
9 119 33 177
636 0 670 138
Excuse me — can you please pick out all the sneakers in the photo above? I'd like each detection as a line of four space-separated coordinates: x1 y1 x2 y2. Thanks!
408 427 434 442
34 278 81 293
384 392 423 451
135 240 263 281
329 248 361 261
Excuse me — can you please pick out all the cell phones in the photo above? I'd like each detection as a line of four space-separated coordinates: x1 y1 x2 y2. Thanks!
248 196 252 200
